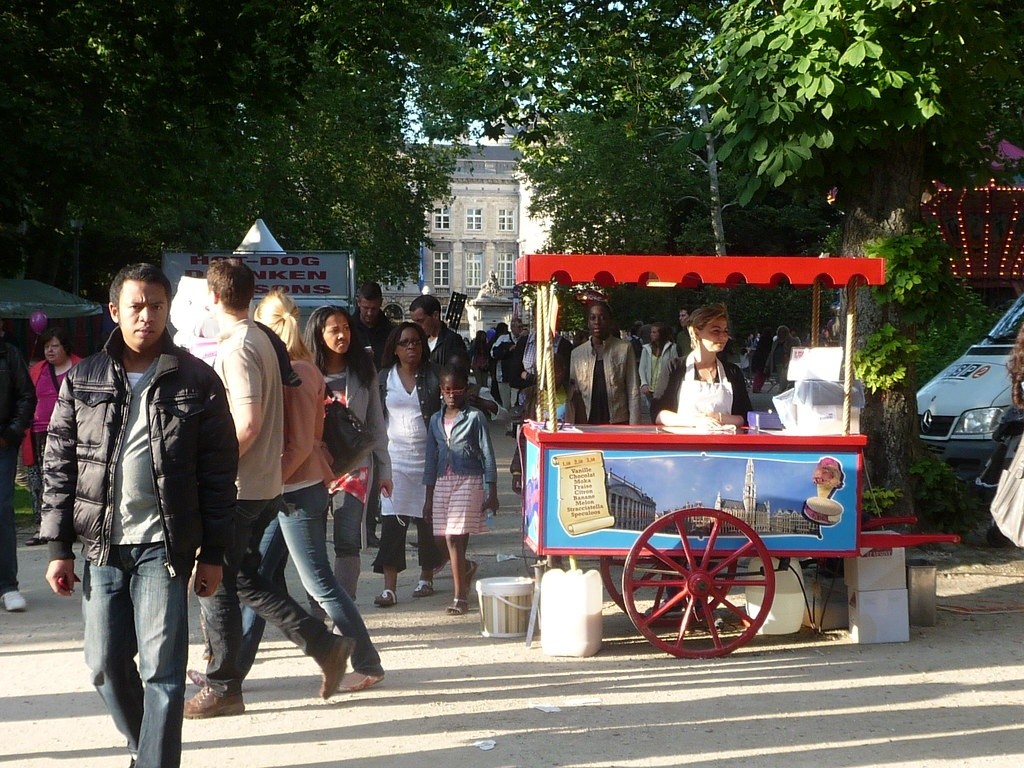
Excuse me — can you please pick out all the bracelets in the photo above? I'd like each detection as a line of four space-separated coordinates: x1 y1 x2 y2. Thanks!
719 412 722 422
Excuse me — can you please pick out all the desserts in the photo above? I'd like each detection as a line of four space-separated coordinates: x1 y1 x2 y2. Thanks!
804 497 843 525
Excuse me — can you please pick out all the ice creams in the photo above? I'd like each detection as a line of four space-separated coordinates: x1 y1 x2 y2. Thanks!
814 457 844 498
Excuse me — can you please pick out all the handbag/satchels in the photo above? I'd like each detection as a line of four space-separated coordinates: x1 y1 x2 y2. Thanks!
322 400 376 480
990 431 1024 547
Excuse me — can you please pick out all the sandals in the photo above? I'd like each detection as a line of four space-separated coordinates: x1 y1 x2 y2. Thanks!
412 580 434 597
25 531 48 545
447 599 469 615
374 589 397 606
466 561 478 599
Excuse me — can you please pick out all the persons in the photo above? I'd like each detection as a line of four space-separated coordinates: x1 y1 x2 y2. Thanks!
565 301 641 426
371 322 449 605
184 259 356 720
990 322 1024 549
332 295 842 549
645 304 752 618
510 354 588 495
0 323 37 612
40 264 240 768
187 289 385 694
24 327 92 546
301 305 393 628
422 368 500 615
486 265 503 295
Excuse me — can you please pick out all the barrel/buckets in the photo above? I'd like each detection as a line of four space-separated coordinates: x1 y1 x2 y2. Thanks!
744 555 806 634
476 577 535 638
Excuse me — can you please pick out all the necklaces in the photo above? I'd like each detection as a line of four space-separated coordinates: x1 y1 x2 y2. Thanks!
698 360 713 380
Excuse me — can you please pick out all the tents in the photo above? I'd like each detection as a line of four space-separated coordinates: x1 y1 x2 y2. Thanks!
0 280 102 322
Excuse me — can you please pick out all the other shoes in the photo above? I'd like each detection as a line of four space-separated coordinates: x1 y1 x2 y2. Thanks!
188 669 205 687
434 559 448 573
338 670 384 692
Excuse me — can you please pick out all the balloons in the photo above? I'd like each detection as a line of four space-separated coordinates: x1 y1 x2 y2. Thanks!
30 311 47 333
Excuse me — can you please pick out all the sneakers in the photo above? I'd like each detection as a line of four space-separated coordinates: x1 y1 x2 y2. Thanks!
0 591 26 611
183 686 245 719
319 634 357 700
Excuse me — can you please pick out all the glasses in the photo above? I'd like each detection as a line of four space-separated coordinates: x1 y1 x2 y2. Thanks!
396 338 423 347
441 385 467 395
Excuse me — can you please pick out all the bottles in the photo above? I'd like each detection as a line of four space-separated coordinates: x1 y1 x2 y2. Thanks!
483 490 494 527
540 568 604 657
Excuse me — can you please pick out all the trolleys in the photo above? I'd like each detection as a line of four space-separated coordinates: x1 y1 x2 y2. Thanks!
512 253 963 661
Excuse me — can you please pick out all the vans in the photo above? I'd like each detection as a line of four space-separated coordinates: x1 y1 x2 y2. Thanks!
914 295 1024 482
